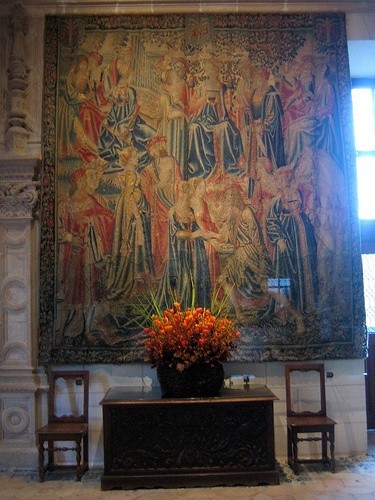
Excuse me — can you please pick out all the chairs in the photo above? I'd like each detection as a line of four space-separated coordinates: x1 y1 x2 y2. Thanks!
36 370 90 482
284 363 337 474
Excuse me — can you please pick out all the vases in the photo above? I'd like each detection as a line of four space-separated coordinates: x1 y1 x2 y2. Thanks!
156 359 226 397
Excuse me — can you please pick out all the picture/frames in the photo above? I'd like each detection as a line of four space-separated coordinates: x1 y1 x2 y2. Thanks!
35 12 369 359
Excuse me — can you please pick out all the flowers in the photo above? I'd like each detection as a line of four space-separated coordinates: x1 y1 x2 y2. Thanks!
130 281 246 360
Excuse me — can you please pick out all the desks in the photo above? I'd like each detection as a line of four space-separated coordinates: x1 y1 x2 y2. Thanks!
99 383 278 491
